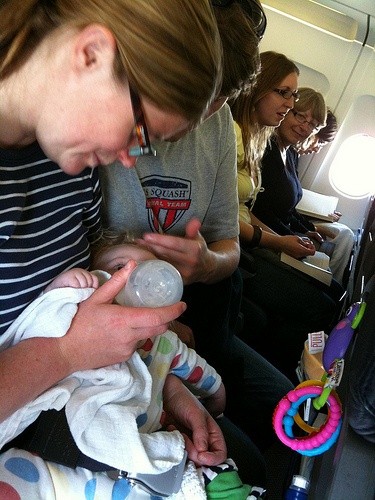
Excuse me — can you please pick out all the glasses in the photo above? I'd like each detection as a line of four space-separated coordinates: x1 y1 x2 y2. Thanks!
291 110 318 131
128 79 156 158
272 87 300 103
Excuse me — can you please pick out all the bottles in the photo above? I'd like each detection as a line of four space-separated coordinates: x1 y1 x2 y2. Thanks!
285 474 312 500
112 259 182 309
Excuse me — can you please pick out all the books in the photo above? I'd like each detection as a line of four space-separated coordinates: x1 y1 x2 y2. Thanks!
294 187 338 223
280 245 333 288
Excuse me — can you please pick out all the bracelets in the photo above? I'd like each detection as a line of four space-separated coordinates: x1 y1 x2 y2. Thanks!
250 223 262 250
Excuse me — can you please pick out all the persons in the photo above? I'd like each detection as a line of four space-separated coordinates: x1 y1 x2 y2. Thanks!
0 1 344 500
